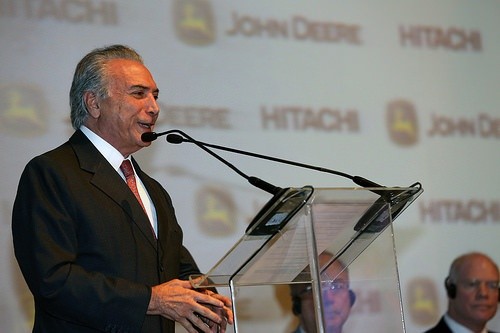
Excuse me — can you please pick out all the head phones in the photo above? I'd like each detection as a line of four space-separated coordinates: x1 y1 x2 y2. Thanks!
445 277 456 298
293 290 356 315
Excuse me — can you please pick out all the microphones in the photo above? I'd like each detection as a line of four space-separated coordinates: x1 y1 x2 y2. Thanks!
141 130 314 237
165 134 418 234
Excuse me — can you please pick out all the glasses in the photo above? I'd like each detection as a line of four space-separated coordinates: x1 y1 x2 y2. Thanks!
299 282 349 294
464 278 500 290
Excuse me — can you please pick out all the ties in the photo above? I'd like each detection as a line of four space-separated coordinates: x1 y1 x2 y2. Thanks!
119 158 157 244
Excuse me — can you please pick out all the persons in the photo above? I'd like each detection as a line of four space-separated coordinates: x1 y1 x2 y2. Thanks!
10 45 233 333
423 251 500 333
290 252 356 333
